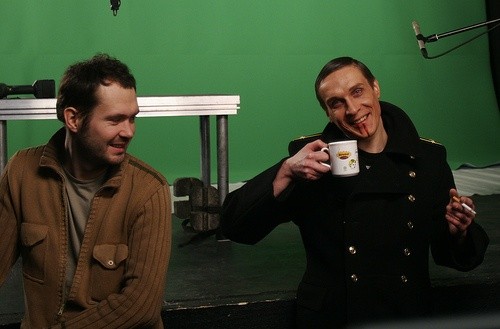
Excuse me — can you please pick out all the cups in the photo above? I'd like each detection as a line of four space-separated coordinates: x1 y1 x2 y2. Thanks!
318 139 361 178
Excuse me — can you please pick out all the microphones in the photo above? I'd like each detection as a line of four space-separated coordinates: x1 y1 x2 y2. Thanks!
412 20 428 59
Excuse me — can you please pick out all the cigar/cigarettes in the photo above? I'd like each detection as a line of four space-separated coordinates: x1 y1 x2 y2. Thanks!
452 196 477 215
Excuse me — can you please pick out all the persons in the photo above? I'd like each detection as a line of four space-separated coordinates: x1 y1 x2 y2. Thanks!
222 57 489 329
0 53 174 329
